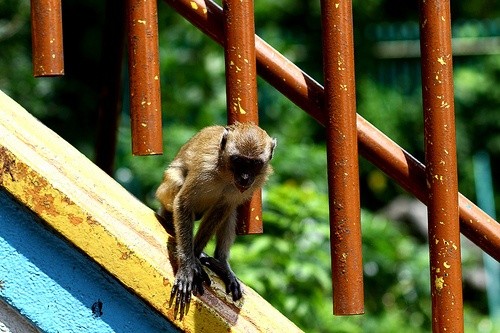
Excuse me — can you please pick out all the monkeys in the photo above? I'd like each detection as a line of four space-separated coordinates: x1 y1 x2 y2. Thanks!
156 121 277 307
